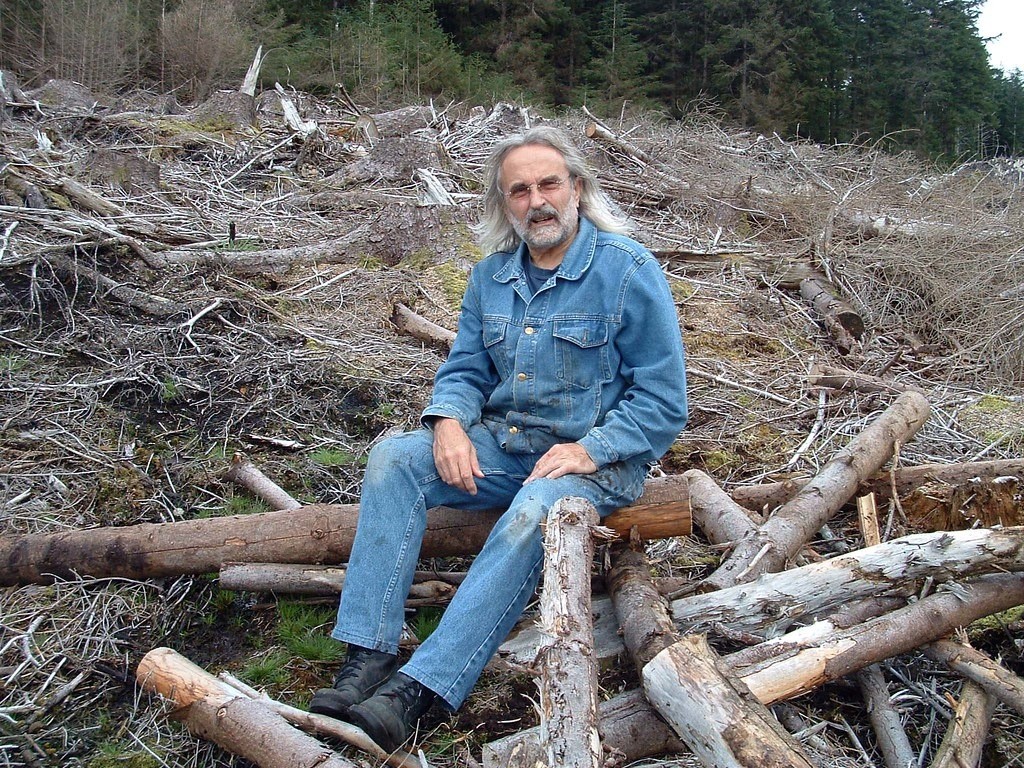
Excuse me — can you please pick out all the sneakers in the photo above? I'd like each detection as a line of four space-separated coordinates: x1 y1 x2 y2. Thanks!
347 673 433 756
308 644 397 719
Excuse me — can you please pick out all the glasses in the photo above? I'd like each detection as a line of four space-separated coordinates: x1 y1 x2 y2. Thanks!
496 174 573 201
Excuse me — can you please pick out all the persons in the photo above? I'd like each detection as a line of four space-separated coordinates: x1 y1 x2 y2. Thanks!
308 125 689 754
